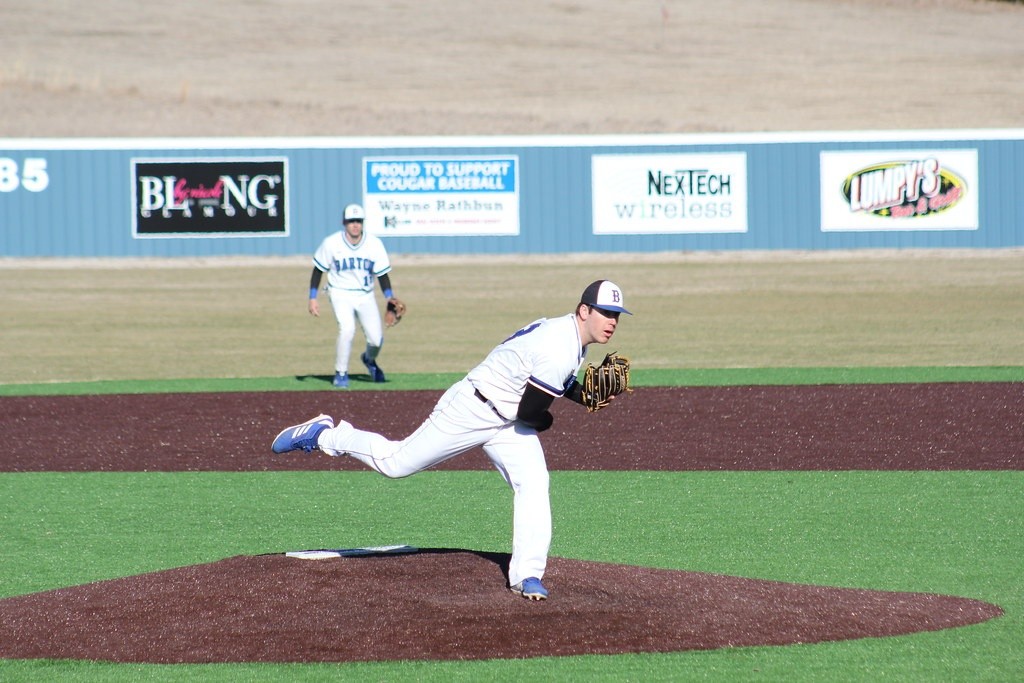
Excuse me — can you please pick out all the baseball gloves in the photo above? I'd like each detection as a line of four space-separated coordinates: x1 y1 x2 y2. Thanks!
383 297 406 327
582 351 635 414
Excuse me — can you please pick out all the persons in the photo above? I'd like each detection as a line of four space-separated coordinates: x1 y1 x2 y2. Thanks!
273 281 634 600
305 202 399 387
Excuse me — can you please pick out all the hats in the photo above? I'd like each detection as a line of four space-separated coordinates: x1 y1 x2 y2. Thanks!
581 280 632 315
343 204 364 222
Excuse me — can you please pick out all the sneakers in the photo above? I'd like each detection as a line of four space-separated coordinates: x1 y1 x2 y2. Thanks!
511 576 549 601
272 415 333 454
360 353 384 381
333 372 349 389
